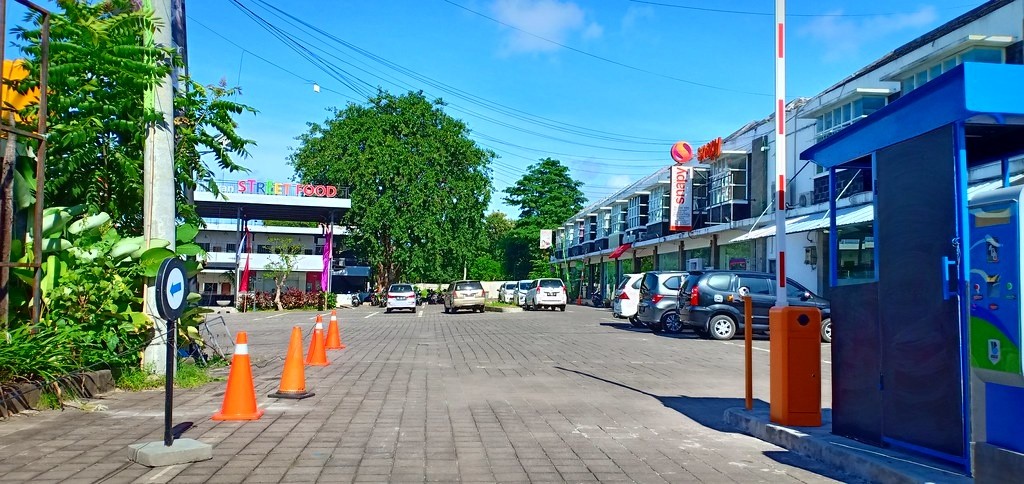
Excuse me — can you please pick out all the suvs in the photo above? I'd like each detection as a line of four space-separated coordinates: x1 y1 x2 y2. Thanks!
443 280 486 313
613 272 649 328
636 270 690 334
675 268 831 345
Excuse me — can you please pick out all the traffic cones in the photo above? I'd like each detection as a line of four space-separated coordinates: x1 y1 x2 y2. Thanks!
323 310 346 350
268 326 316 400
305 315 330 366
577 295 582 305
211 330 264 422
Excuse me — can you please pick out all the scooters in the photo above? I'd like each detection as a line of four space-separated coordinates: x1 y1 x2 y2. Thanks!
416 288 445 306
348 289 362 307
369 287 387 308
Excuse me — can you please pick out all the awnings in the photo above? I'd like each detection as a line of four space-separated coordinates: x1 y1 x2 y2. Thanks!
728 160 874 243
608 244 631 259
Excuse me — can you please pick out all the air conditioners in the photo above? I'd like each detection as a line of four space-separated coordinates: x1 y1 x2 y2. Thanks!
769 177 791 211
797 191 813 208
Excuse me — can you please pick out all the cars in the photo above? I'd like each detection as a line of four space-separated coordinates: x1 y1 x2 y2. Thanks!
496 282 517 304
385 284 417 313
524 277 567 311
513 280 542 309
